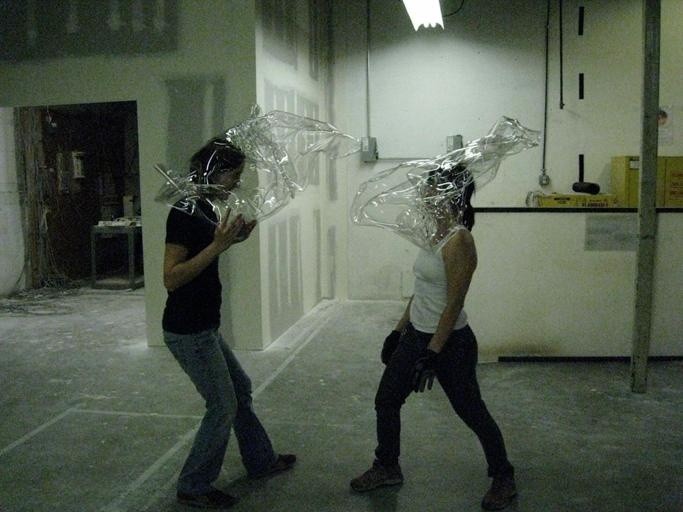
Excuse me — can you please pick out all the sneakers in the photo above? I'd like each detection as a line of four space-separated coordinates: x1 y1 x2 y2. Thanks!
248 454 296 479
177 487 240 508
351 466 403 492
482 477 517 511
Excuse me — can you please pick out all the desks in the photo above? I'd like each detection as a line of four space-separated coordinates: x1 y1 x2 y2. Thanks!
90 225 143 290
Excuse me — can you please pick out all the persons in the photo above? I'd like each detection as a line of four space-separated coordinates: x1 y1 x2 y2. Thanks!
350 164 517 512
162 137 296 509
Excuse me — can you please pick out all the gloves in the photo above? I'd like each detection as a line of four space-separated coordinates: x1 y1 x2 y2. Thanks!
410 348 440 393
382 330 401 365
